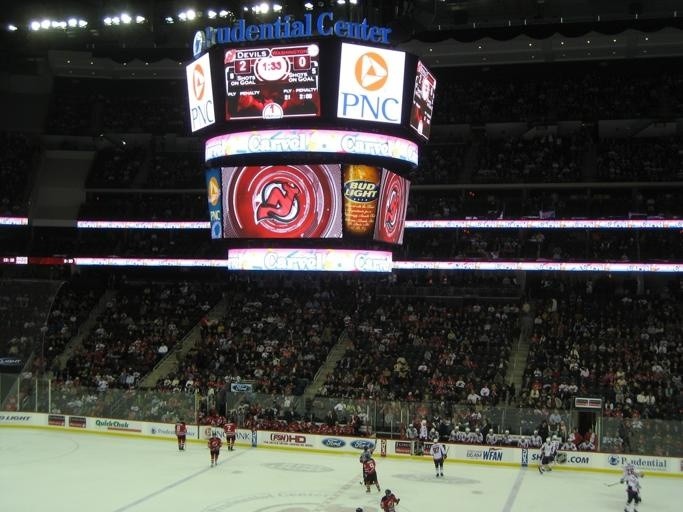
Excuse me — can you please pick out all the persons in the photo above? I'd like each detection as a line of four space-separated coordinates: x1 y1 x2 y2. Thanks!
208 432 222 466
304 73 523 447
224 419 236 451
363 455 380 491
521 70 682 456
430 439 446 478
621 466 641 511
360 446 375 485
538 438 556 473
47 93 304 433
1 131 52 413
381 489 399 512
175 418 187 450
